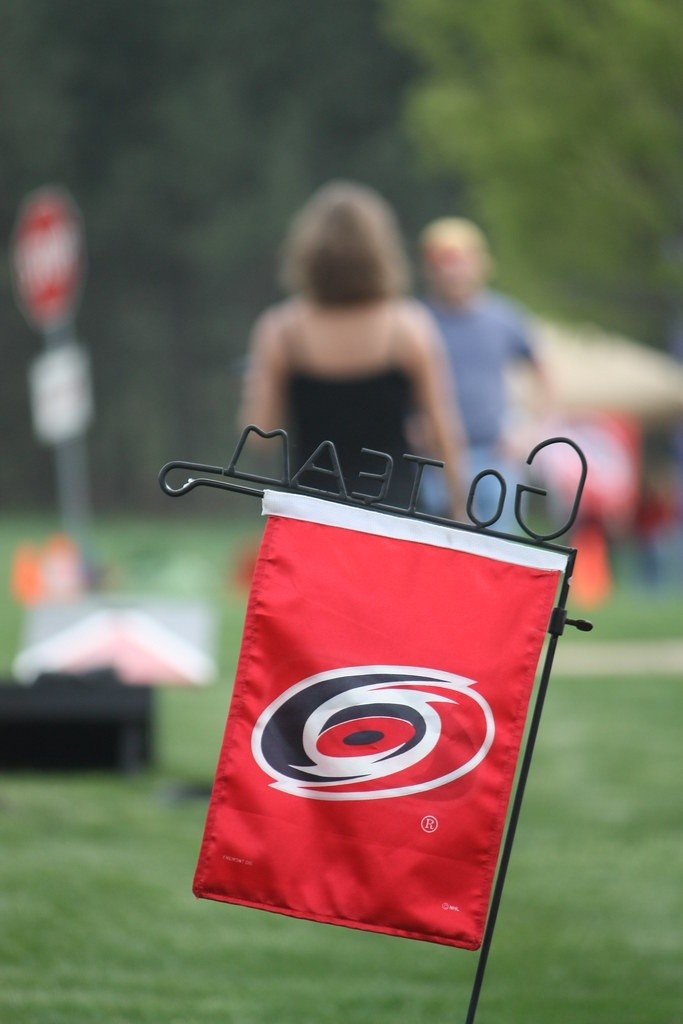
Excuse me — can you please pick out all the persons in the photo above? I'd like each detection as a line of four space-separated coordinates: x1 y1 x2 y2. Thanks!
413 215 563 534
238 183 472 528
